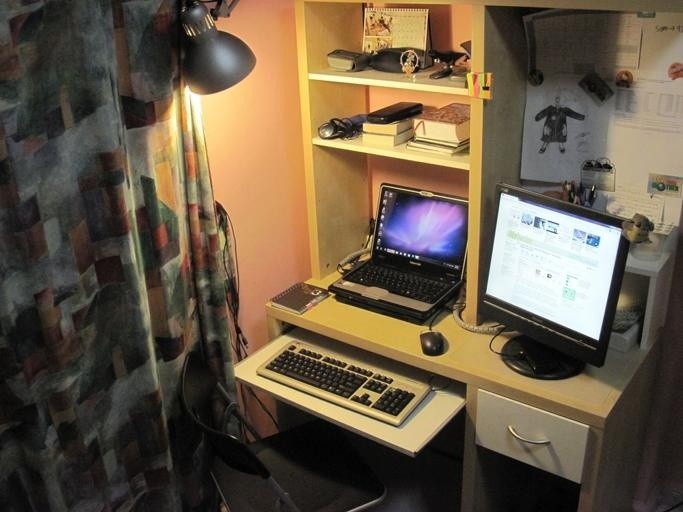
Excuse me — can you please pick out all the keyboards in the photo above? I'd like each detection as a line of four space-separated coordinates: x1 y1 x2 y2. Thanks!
257 342 431 427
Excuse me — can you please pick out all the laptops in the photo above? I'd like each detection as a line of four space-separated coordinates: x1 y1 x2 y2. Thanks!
328 182 470 318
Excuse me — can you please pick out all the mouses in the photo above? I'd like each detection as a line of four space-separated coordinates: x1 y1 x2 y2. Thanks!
420 330 442 355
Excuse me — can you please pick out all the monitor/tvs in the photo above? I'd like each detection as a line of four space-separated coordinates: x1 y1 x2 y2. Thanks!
480 181 633 381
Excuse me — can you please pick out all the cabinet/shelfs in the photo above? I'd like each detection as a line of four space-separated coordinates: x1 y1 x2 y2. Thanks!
297 2 475 320
474 386 592 485
621 245 671 350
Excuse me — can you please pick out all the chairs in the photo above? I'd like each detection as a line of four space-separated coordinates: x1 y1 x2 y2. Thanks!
179 339 389 512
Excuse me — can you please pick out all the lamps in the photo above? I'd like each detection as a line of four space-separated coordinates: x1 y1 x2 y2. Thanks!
176 1 258 96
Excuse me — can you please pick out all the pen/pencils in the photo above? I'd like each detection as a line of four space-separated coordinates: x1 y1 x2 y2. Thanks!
561 180 597 209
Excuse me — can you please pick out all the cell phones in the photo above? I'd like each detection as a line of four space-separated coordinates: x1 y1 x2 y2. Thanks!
520 343 556 373
367 102 423 123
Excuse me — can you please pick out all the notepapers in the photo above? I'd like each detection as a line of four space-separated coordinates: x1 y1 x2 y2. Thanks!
467 72 495 100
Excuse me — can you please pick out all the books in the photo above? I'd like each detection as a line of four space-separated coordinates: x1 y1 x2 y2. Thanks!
361 102 469 157
269 281 329 315
